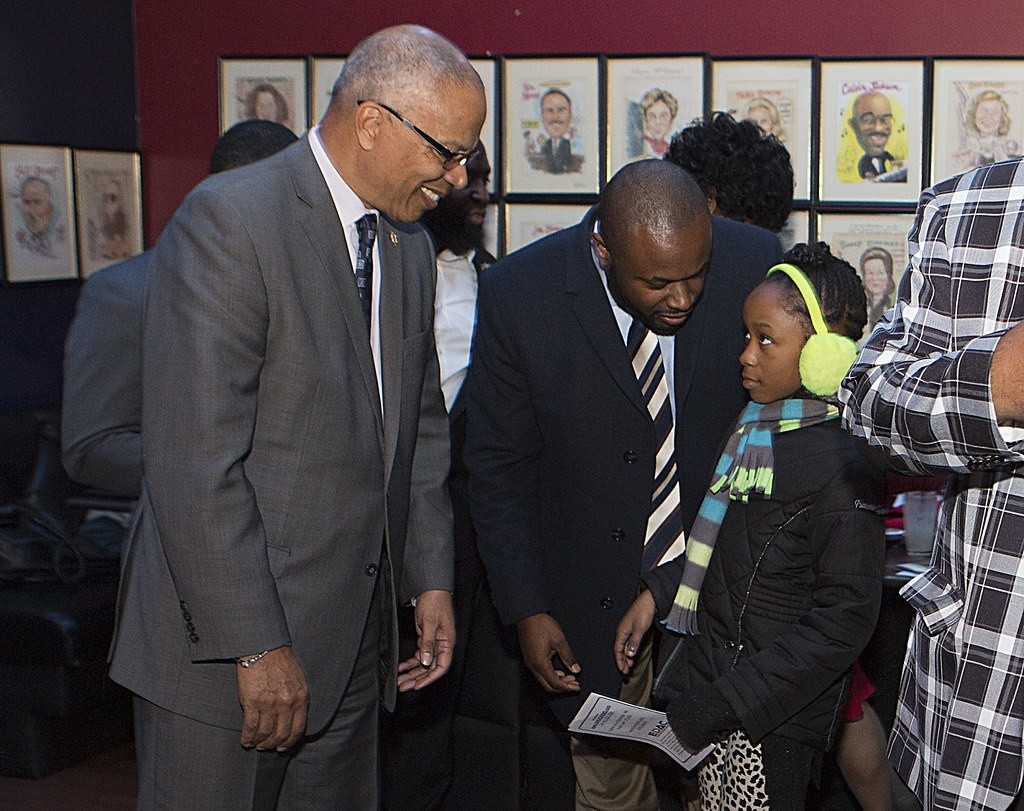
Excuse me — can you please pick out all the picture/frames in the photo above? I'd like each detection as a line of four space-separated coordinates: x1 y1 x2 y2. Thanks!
463 54 501 201
216 53 307 137
309 53 348 125
73 148 146 277
929 54 1023 187
0 144 79 284
709 56 814 204
501 205 595 258
484 201 502 259
606 53 709 182
503 52 603 203
817 56 927 208
768 206 815 256
817 210 921 346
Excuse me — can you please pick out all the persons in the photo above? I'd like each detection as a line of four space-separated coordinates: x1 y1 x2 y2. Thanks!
377 136 526 811
662 237 887 811
450 157 783 811
56 115 301 503
841 159 1023 811
666 109 796 235
100 27 487 811
828 662 923 811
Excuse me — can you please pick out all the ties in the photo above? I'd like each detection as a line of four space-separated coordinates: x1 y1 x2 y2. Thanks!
627 312 686 573
354 213 378 348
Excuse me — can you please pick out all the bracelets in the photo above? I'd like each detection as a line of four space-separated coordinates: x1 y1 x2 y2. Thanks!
234 651 271 668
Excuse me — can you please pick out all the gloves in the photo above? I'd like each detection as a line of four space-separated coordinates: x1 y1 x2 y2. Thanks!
667 683 742 756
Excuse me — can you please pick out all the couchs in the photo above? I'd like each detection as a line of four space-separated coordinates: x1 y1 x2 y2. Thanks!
0 407 135 780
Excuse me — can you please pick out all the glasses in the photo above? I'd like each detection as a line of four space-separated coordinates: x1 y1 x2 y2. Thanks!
355 99 481 170
